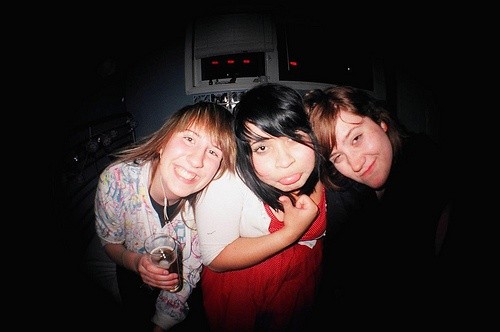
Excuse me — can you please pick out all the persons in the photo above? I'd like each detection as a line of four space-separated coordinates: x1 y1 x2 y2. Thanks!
92 82 454 332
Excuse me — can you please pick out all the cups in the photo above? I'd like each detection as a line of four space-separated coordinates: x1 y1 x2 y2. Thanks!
144 233 183 293
